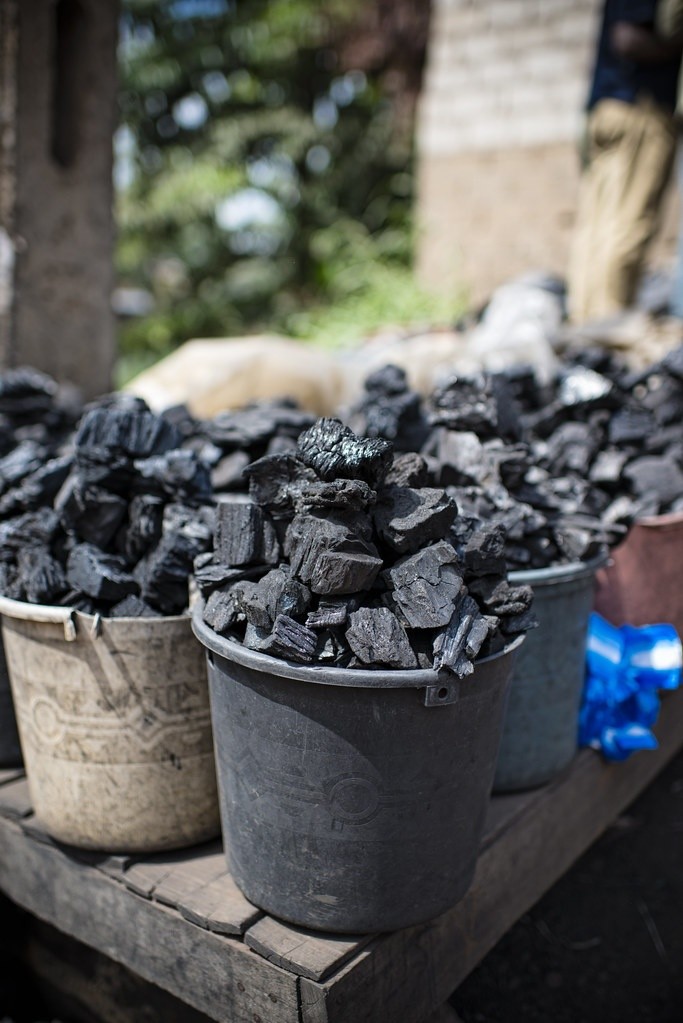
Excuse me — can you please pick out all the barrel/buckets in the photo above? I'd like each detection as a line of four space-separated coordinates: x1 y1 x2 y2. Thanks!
191 598 526 935
595 510 683 646
0 596 224 855
491 546 611 789
